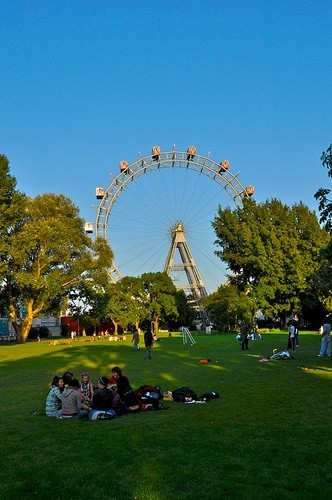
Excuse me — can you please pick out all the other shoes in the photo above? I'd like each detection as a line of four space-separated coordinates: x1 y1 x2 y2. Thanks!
317 354 323 357
327 354 331 357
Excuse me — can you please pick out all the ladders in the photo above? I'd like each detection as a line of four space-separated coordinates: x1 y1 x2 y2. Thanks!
182 327 197 344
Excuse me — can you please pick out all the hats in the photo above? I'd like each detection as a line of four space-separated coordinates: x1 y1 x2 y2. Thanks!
100 376 109 386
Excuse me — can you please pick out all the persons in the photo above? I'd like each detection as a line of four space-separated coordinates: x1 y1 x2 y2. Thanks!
72 331 76 340
144 328 153 358
287 321 299 352
133 329 140 350
169 333 173 340
104 330 126 341
153 335 159 341
45 367 139 419
237 322 257 351
317 318 332 356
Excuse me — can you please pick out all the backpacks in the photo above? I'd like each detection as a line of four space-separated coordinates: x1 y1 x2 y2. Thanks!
172 387 198 402
135 384 162 399
